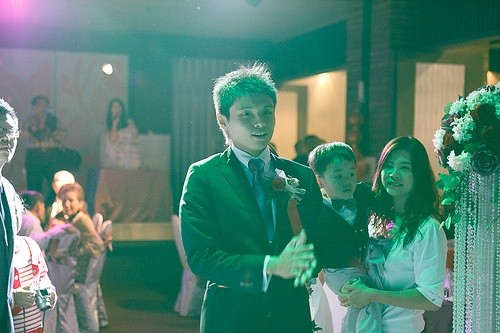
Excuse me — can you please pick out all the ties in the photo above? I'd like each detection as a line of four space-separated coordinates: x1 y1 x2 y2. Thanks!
248 157 275 242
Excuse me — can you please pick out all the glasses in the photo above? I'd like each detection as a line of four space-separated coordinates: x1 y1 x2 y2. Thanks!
0 127 23 138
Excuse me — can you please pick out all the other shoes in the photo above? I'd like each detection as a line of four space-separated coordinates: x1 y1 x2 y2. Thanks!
99 319 109 327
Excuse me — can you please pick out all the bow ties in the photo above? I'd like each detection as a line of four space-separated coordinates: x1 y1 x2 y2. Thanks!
331 198 357 211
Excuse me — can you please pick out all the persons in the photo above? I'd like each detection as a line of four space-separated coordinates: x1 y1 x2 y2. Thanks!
94 97 143 225
58 184 109 333
7 187 72 333
308 143 390 332
0 99 25 333
41 169 76 235
291 133 332 173
180 60 323 333
42 188 82 333
22 94 60 149
335 136 448 333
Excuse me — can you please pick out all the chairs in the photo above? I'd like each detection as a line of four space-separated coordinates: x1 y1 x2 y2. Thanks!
43 212 114 333
171 214 206 317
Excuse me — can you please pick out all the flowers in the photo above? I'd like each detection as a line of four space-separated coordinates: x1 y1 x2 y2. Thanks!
262 168 306 207
432 82 500 231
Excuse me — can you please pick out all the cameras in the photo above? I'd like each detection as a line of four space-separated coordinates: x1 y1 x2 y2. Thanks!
26 287 52 312
